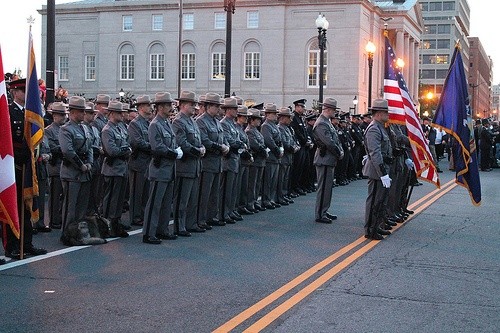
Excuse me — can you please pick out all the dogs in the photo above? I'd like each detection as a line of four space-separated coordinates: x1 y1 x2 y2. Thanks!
59 214 130 247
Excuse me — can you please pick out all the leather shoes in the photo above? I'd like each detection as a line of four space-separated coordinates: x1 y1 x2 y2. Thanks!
395 216 404 222
388 219 397 225
365 232 382 239
19 246 47 254
114 172 368 244
405 208 414 214
384 224 392 229
5 251 27 258
323 211 337 219
316 216 332 223
380 229 391 235
398 213 408 219
401 211 409 216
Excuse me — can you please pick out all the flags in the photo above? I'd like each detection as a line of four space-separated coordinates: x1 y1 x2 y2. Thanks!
384 30 440 189
0 47 20 241
22 32 45 226
430 44 482 206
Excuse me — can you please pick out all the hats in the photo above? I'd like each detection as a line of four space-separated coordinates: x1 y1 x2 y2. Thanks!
7 79 26 89
367 99 390 110
46 91 372 121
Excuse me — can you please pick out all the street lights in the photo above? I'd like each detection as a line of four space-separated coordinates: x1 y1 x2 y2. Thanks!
366 40 377 113
469 81 491 116
314 11 329 113
352 95 358 115
483 108 496 118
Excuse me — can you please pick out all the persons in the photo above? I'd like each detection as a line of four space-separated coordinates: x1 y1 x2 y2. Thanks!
311 97 344 224
362 98 393 240
0 73 500 266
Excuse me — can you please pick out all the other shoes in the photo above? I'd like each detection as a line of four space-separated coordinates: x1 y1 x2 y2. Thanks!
481 167 492 171
37 226 51 231
410 183 419 186
419 183 423 185
49 224 61 229
31 228 38 233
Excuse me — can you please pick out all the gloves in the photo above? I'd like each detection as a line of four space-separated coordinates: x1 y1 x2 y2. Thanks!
381 174 392 188
176 146 183 159
405 158 414 170
362 155 368 165
